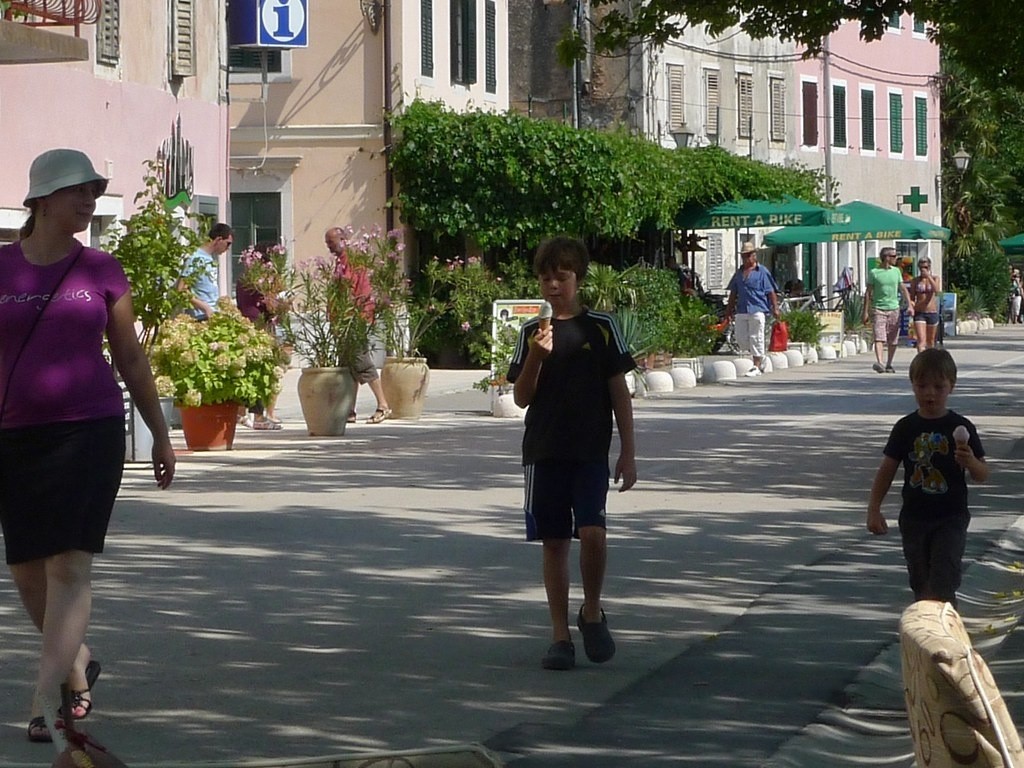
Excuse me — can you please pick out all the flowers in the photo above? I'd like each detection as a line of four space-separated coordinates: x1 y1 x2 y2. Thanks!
237 235 387 368
338 221 502 359
144 295 290 407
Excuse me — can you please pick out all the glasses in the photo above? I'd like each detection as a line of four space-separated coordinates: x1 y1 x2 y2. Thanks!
222 236 232 246
884 254 897 258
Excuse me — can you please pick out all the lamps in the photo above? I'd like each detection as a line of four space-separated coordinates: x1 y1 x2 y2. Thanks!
668 122 695 148
935 141 971 188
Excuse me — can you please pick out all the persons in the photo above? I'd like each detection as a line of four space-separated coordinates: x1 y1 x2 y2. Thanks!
0 148 176 742
236 241 282 430
177 223 234 323
504 236 637 670
325 227 393 424
909 256 940 354
866 348 988 611
862 247 915 373
1006 263 1024 324
723 242 780 377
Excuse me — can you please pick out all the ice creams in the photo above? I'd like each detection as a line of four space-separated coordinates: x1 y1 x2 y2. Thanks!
953 425 970 471
538 301 552 331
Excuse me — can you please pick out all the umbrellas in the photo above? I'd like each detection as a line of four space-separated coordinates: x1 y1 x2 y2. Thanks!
670 192 835 289
998 234 1024 255
760 201 951 295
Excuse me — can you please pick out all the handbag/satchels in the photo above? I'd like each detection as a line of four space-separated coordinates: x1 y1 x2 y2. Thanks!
769 316 787 351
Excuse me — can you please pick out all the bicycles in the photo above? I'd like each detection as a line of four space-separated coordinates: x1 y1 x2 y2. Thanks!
781 284 854 323
726 319 742 356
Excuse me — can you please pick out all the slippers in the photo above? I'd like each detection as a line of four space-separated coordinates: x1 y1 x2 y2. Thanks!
68 661 101 718
28 683 66 741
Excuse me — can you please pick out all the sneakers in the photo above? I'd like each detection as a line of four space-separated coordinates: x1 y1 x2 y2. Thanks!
745 366 763 376
885 365 895 373
872 363 885 373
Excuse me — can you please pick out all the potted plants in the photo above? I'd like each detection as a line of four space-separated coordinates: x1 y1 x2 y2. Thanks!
99 139 995 467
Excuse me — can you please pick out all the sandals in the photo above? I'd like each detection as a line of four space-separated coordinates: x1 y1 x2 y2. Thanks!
542 640 575 670
367 407 392 423
578 603 615 663
237 413 254 428
254 418 281 430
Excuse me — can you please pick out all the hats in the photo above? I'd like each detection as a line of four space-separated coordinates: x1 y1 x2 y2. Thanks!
23 149 108 207
737 242 757 253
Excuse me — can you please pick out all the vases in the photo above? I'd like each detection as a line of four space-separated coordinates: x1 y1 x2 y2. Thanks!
381 357 430 420
298 368 357 436
179 401 238 451
